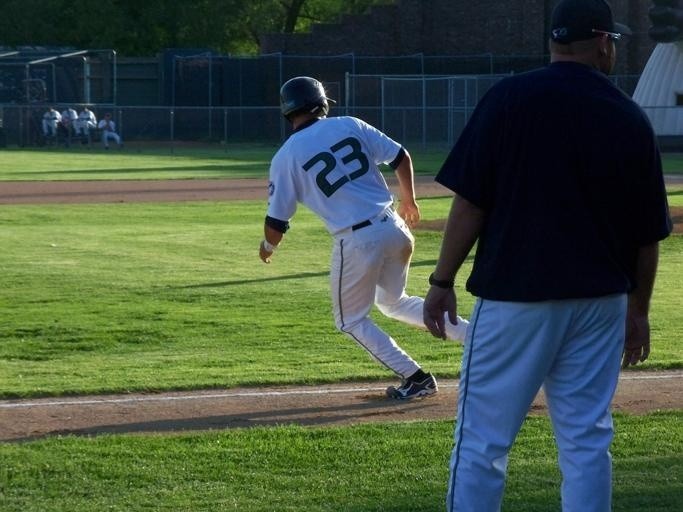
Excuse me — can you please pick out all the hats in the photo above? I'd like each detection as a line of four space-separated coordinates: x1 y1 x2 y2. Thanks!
547 1 634 43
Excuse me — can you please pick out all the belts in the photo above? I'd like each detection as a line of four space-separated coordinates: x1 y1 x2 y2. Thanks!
353 220 372 231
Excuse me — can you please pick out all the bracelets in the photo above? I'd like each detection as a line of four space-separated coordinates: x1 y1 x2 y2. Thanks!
428 274 454 288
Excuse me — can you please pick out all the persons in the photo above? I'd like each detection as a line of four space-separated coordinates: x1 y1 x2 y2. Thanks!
422 0 673 512
79 106 98 135
61 106 82 137
42 105 62 135
259 77 470 400
98 112 121 148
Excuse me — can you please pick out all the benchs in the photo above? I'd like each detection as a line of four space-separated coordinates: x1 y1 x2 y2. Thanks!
35 120 102 151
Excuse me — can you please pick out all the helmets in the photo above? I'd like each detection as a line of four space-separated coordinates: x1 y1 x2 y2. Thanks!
279 77 329 117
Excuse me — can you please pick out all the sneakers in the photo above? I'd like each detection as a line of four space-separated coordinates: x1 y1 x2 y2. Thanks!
385 373 438 401
